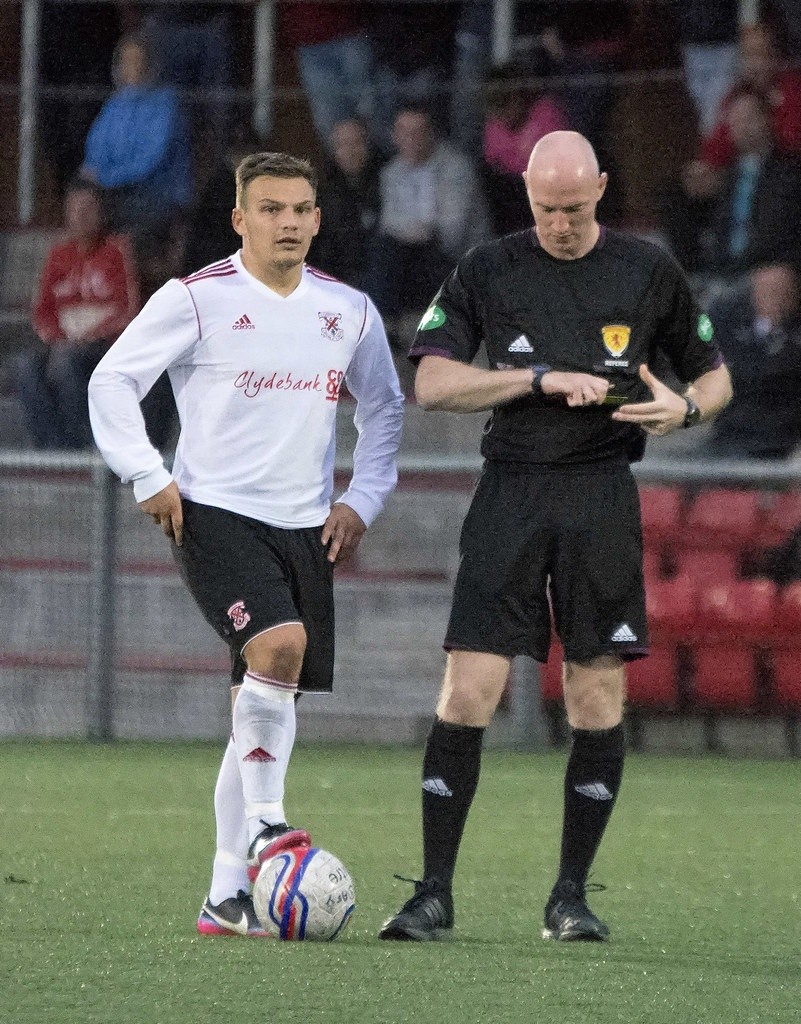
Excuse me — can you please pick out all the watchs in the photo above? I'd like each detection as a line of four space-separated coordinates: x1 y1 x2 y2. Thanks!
532 364 553 397
682 396 700 429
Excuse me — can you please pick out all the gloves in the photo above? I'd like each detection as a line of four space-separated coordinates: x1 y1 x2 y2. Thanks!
678 394 701 429
531 362 552 395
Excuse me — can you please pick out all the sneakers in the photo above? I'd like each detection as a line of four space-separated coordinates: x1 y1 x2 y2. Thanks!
379 874 454 941
197 888 269 936
545 872 610 942
246 819 311 883
17 429 91 451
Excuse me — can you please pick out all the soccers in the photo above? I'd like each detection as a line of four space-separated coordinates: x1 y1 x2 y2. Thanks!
254 846 356 939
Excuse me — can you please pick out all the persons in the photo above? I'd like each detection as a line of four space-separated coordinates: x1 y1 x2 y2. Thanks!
378 130 737 941
89 154 405 938
14 0 800 454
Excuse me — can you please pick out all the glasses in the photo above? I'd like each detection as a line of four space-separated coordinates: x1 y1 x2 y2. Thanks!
330 135 370 147
728 120 767 131
